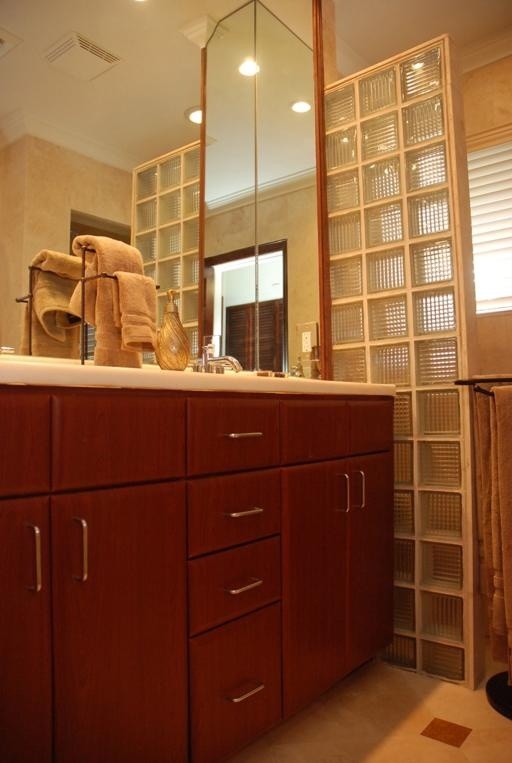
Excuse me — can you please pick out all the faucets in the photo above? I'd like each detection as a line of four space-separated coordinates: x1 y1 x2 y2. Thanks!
199 343 242 373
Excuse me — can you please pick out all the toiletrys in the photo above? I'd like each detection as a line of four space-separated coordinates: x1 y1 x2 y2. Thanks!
153 289 191 372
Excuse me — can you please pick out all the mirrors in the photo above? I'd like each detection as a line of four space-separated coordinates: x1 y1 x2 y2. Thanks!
0 1 333 380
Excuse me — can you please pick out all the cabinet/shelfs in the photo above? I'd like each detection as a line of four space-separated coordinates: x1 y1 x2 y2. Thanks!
182 393 284 763
1 388 189 760
282 397 396 723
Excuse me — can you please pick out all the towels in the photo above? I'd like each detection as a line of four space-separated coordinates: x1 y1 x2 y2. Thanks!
470 381 511 689
72 233 158 369
18 248 90 360
